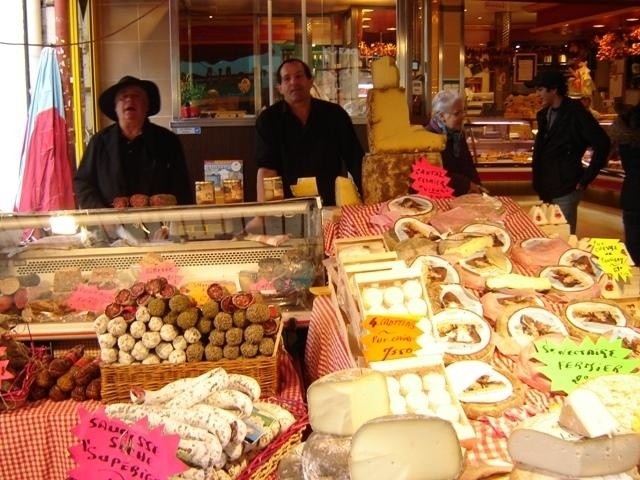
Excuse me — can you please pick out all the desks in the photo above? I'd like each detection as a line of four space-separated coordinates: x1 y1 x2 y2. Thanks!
315 190 639 480
0 341 302 480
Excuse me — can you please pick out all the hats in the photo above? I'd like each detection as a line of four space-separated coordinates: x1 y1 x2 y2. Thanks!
98 75 161 121
525 70 566 89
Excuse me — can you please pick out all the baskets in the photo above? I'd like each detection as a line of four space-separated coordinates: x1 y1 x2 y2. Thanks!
96 318 287 407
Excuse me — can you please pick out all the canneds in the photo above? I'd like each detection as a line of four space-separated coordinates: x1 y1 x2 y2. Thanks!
263 176 283 201
223 179 243 203
195 181 215 204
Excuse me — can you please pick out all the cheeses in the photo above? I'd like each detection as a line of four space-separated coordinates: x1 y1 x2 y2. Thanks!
302 193 639 480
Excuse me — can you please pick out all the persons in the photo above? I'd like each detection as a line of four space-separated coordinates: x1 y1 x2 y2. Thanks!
232 57 366 243
524 66 611 235
423 88 485 197
73 75 196 243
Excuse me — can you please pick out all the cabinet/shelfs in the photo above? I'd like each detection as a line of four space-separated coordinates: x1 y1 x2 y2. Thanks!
461 114 539 195
580 119 624 209
1 198 323 339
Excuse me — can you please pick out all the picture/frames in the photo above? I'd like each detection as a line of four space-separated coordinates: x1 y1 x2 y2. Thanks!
513 52 538 84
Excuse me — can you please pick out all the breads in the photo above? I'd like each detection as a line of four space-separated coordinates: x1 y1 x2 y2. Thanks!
363 55 448 204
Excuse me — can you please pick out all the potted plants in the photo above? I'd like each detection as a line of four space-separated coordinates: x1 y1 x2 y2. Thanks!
179 79 206 120
